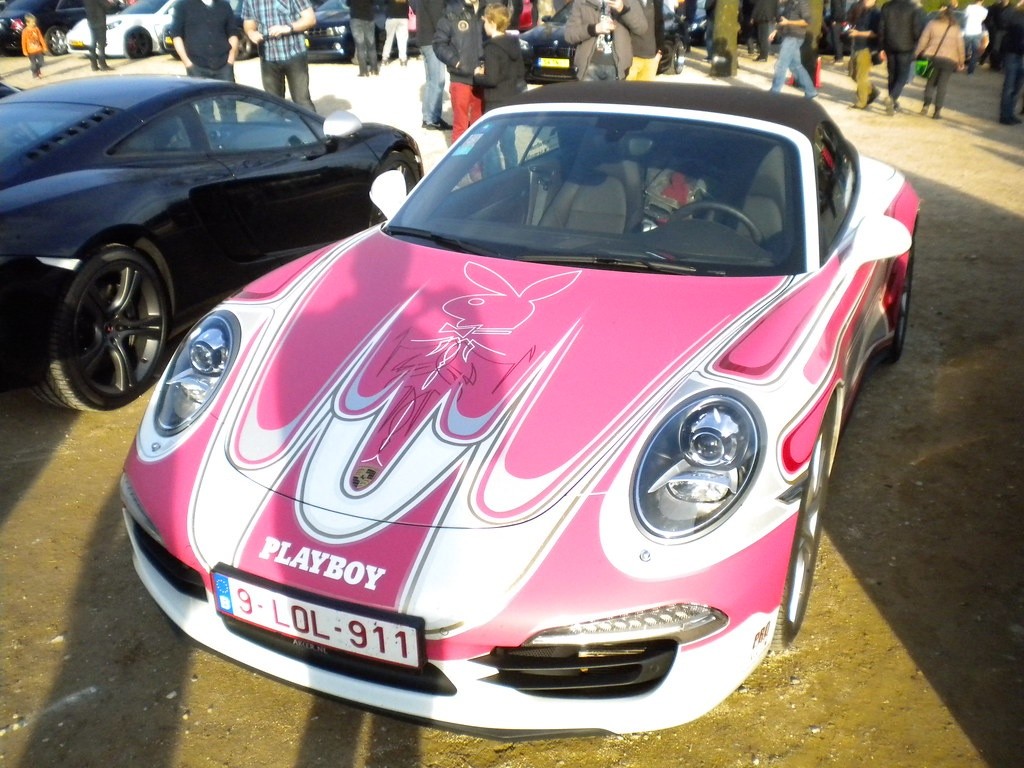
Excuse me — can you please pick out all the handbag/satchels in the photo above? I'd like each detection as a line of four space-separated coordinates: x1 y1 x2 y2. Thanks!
870 53 884 65
915 56 937 78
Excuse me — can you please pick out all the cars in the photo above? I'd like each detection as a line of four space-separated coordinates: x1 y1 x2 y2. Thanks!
0 0 127 56
305 0 419 67
518 0 693 82
926 11 989 63
687 0 707 45
117 77 922 742
156 0 258 61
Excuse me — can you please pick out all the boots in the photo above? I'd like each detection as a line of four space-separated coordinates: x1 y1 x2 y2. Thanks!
919 102 932 115
932 106 942 118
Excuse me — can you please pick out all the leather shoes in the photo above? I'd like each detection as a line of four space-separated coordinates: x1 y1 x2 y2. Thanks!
423 120 453 130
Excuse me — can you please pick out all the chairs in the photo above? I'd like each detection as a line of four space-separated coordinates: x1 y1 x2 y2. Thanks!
538 148 638 236
737 143 788 243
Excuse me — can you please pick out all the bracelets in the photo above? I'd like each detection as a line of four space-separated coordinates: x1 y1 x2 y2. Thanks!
285 23 293 33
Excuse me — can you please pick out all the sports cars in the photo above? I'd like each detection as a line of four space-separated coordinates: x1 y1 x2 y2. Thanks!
0 73 425 411
64 0 177 60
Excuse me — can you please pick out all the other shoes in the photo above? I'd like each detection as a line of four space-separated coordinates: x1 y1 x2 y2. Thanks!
744 50 757 56
803 92 818 99
32 74 43 81
883 98 900 118
380 59 390 66
1000 117 1022 125
868 90 881 105
848 105 866 110
93 66 114 72
830 59 844 65
358 71 378 77
702 57 711 62
957 64 974 75
755 56 767 62
399 60 407 67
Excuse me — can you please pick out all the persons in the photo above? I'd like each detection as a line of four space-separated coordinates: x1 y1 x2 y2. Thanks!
169 0 240 123
564 0 658 82
83 0 117 71
380 0 409 66
346 0 379 76
242 0 317 113
473 4 528 114
432 0 488 144
410 0 453 130
480 0 523 31
21 12 46 78
675 0 1024 125
